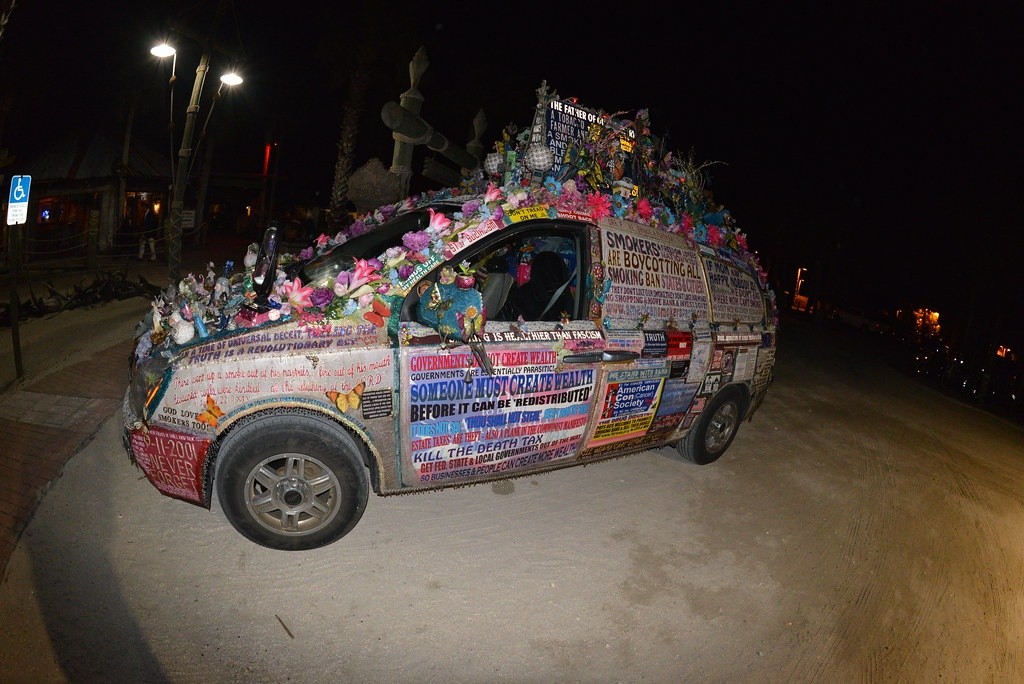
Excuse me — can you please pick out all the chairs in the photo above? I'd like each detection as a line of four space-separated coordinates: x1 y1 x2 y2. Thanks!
507 251 574 321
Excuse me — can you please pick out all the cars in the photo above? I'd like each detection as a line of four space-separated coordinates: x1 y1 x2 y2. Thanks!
119 108 778 556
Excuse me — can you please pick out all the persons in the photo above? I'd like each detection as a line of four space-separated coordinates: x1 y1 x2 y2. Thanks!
137 200 157 261
507 252 575 322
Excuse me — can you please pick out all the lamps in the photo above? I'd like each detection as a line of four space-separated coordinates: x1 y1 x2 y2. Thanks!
220 65 244 88
150 40 176 59
